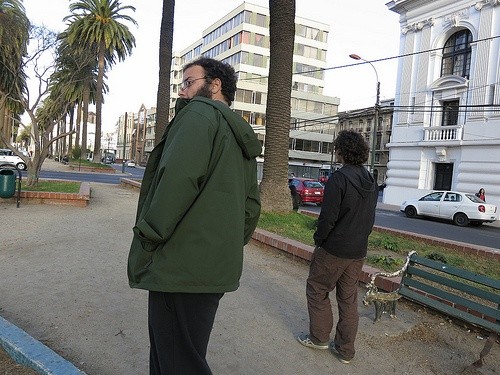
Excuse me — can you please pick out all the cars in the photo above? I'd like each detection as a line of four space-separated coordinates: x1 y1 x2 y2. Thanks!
287 176 325 207
400 190 498 227
0 147 31 170
123 160 136 168
319 176 328 184
102 156 115 164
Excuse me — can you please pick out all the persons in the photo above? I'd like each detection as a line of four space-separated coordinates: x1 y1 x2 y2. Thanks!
127 59 263 375
476 188 486 201
297 129 378 363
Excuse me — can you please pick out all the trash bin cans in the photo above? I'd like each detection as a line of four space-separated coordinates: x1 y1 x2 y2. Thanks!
0 168 15 198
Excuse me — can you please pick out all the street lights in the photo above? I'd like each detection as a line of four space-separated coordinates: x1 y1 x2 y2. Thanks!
349 52 381 179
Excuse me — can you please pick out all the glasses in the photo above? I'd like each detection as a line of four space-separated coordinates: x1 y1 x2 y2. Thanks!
179 76 213 91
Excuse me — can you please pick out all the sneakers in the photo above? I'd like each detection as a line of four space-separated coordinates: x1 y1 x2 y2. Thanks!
298 333 329 350
329 341 352 364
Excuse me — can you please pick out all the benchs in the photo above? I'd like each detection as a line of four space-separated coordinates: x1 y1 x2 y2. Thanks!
363 250 500 340
